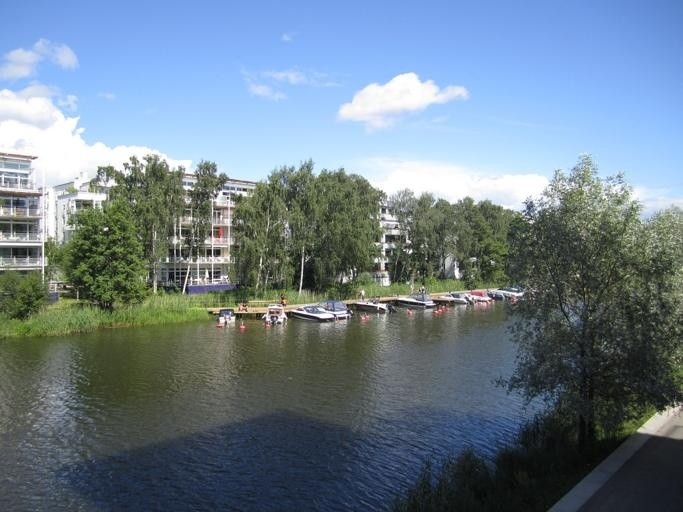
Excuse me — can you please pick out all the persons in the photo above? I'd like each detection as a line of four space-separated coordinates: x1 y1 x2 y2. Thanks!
281 294 287 307
361 288 365 301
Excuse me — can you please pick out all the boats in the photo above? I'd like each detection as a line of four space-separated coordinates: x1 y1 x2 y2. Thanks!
262 303 288 324
216 308 236 325
397 284 436 308
442 287 527 306
318 300 353 319
355 296 396 314
289 303 335 323
